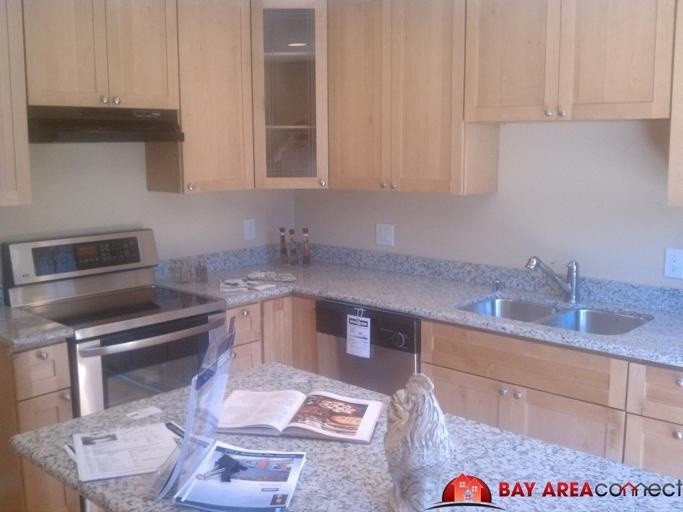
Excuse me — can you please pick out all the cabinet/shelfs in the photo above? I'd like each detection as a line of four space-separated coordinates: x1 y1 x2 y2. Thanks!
418 284 628 463
252 0 325 190
0 2 31 209
24 1 180 107
624 312 683 477
231 262 295 371
1 316 83 512
324 1 498 195
178 3 253 194
467 1 676 119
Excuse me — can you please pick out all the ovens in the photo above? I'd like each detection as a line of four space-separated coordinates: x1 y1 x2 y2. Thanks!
57 316 236 420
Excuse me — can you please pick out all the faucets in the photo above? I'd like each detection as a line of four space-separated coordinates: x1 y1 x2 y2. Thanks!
525 255 580 306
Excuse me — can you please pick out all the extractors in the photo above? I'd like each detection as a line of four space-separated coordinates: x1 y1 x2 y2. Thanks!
5 227 229 342
26 105 184 143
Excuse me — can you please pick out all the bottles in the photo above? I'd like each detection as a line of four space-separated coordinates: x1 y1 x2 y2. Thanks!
289 229 298 267
279 227 288 265
302 228 309 266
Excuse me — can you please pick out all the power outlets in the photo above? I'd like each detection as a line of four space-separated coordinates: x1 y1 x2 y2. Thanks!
662 246 680 279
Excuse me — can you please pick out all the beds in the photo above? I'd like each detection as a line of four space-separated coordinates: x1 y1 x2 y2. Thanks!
12 359 682 511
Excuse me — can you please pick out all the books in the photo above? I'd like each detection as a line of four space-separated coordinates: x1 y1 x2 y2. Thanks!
173 442 306 508
212 389 383 444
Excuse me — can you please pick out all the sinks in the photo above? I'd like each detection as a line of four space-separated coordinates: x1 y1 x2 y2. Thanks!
541 309 649 337
458 296 563 324
